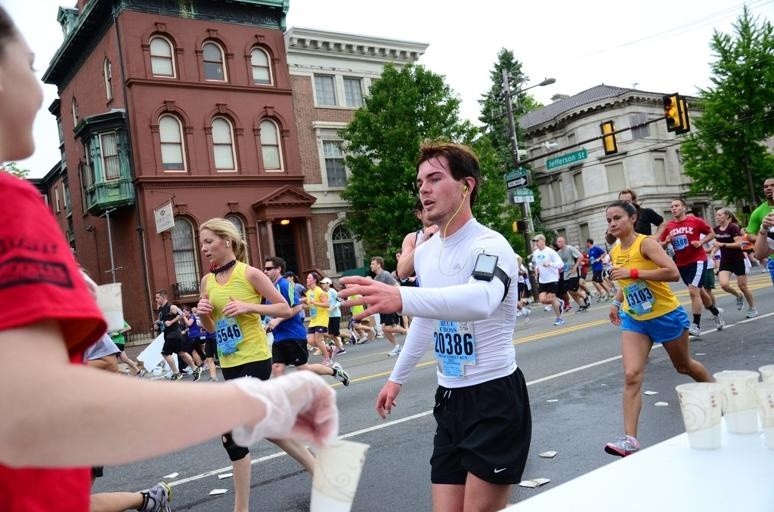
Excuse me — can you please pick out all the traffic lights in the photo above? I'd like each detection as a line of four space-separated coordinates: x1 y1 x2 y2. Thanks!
663 94 680 129
677 95 691 132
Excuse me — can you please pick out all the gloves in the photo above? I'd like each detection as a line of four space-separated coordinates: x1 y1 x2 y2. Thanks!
225 369 344 452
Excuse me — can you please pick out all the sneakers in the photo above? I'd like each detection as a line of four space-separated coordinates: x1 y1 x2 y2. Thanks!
688 323 701 337
309 325 380 365
712 307 724 331
603 434 640 457
735 291 744 310
136 370 144 378
387 345 401 357
515 290 615 326
144 480 172 511
745 308 758 318
170 372 183 381
191 364 203 382
332 361 350 386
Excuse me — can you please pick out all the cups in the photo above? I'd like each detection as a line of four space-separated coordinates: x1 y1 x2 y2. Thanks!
307 438 372 512
674 362 774 452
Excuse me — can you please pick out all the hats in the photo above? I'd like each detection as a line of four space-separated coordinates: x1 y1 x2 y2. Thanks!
530 233 545 242
319 277 332 284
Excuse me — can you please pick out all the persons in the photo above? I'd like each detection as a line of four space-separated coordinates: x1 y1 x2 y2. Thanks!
0 3 340 512
77 266 171 512
606 200 715 458
107 177 774 385
196 218 318 510
336 142 533 511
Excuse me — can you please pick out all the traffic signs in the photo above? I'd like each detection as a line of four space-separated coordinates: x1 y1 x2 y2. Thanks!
504 168 527 182
545 148 587 170
506 174 528 190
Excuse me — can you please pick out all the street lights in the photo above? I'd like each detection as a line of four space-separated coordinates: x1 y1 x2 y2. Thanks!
502 76 557 305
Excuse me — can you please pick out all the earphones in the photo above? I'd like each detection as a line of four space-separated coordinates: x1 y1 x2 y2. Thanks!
227 241 230 248
464 185 469 192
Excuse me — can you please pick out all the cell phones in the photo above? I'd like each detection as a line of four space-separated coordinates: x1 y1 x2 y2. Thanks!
471 254 498 280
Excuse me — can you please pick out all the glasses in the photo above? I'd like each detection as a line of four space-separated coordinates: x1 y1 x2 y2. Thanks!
263 266 277 271
